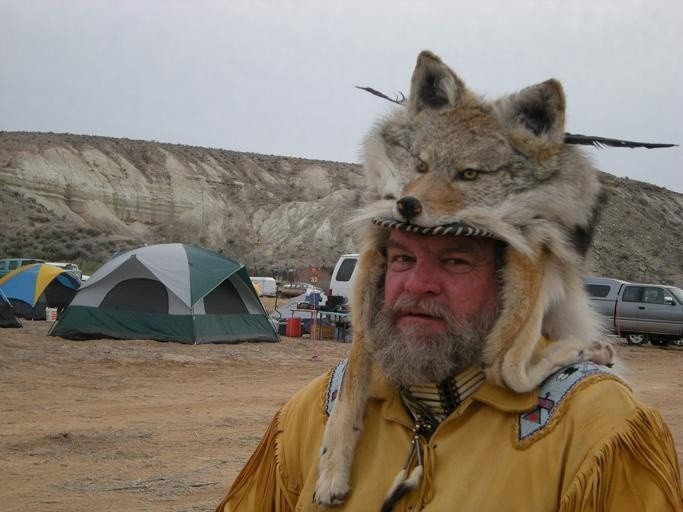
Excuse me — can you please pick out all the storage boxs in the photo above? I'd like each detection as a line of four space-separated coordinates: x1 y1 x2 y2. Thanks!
310 324 335 340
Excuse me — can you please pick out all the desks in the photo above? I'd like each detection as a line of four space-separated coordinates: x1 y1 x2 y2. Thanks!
289 308 351 342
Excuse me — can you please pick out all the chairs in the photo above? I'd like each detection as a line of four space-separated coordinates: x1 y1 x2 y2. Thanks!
647 293 655 303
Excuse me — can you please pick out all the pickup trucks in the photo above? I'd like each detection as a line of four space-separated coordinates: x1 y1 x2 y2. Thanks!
583 277 682 347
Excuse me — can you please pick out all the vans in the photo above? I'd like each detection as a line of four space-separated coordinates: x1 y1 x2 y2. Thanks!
248 276 275 297
327 254 361 312
0 259 45 280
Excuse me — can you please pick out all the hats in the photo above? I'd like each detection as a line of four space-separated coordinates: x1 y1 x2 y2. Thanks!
345 50 606 355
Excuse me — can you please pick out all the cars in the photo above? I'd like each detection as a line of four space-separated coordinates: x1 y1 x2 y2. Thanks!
277 282 315 299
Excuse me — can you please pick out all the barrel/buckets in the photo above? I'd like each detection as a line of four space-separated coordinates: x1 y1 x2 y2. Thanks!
310 291 320 306
46 307 57 322
287 318 301 337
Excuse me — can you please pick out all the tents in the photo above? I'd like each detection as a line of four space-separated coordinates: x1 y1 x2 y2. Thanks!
42 238 284 346
0 280 23 326
0 258 84 324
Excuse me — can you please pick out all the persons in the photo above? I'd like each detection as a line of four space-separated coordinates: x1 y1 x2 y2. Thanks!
213 222 682 510
